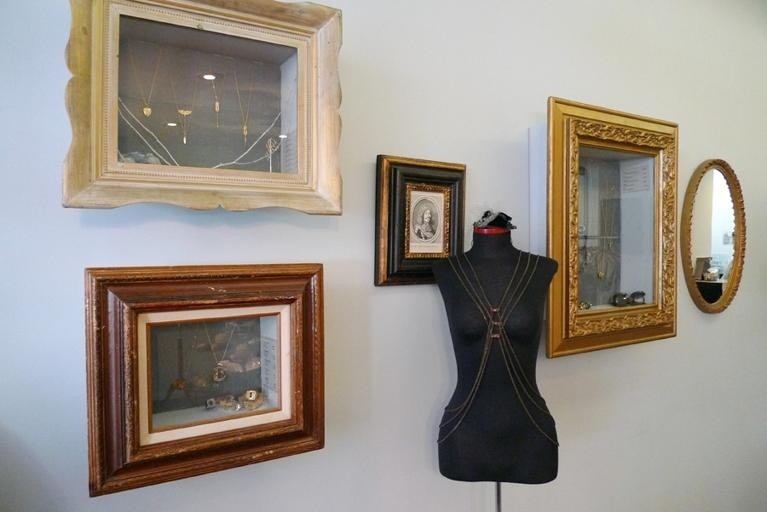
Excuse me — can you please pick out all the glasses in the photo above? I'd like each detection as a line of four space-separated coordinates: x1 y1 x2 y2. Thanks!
611 291 646 306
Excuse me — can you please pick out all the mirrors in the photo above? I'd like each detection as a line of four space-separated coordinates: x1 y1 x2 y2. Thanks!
684 157 750 316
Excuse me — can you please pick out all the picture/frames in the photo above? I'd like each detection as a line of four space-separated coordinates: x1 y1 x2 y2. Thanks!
372 151 468 290
61 0 346 219
537 94 682 361
83 258 330 500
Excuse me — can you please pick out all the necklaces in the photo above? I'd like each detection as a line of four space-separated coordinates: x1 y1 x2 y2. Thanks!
202 321 238 381
128 40 162 118
206 54 230 128
600 191 615 249
589 179 607 279
231 58 255 144
164 46 202 144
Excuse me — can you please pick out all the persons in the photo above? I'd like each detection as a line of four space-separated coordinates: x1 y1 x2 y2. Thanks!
432 212 558 484
414 205 436 241
